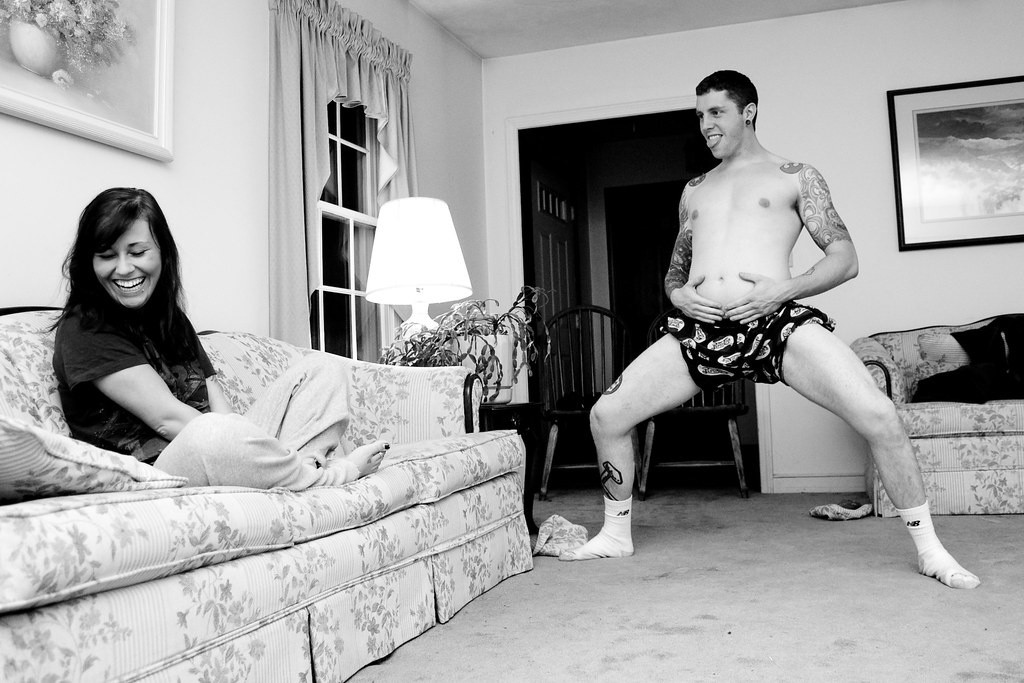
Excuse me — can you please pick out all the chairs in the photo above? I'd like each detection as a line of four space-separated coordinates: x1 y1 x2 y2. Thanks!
638 308 749 502
538 306 642 501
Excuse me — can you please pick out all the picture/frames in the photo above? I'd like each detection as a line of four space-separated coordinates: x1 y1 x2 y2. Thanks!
886 75 1024 252
0 0 176 164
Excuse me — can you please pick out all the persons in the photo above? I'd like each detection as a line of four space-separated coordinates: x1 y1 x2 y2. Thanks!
42 187 390 491
558 69 981 589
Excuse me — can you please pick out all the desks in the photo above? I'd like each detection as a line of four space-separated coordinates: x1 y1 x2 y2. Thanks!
479 402 551 535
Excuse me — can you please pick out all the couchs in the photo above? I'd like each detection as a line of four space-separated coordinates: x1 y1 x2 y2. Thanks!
849 313 1024 519
0 306 534 683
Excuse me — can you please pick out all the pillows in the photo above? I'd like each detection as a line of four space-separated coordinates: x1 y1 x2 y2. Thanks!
917 332 1009 379
0 416 189 502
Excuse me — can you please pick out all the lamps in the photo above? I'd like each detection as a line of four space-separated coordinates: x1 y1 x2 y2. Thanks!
364 196 473 366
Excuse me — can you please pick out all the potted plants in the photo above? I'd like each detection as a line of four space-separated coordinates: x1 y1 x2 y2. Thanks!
376 285 558 404
